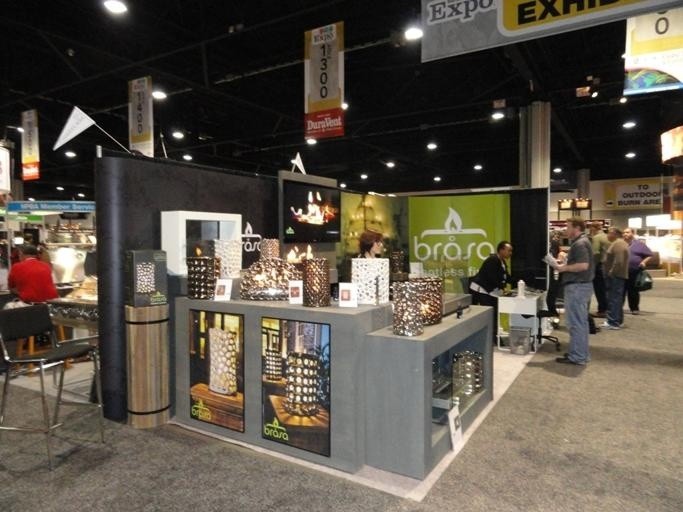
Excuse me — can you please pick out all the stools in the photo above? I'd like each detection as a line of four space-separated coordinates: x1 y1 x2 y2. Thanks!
15 300 71 376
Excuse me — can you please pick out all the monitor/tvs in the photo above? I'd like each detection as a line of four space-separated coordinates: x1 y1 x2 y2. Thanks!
282 179 342 244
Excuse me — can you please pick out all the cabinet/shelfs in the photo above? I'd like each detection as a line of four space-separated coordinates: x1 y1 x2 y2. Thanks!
364 304 494 479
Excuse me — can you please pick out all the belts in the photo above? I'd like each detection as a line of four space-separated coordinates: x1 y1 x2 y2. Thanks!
570 281 590 283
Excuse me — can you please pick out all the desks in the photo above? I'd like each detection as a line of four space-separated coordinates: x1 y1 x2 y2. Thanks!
497 287 546 353
46 290 99 398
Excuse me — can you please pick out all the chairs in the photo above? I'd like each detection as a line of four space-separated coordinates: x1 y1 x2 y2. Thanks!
0 304 104 470
520 279 561 351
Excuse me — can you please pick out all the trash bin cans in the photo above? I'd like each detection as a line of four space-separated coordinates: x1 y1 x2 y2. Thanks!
510 326 531 355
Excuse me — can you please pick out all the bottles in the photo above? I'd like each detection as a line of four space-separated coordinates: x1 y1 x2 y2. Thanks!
457 302 462 319
517 280 525 297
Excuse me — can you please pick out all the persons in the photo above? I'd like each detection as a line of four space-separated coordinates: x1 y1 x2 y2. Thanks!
19 233 50 263
353 231 384 258
469 241 518 307
7 245 60 303
552 216 596 366
589 220 653 330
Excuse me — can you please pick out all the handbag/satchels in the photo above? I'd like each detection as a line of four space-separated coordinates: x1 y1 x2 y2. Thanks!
634 271 652 291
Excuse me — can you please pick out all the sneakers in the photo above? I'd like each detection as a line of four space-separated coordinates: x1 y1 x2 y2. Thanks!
589 311 607 318
556 353 574 365
599 321 625 330
632 310 640 314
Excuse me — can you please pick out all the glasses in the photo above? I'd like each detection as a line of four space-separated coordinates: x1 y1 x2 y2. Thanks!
377 240 382 247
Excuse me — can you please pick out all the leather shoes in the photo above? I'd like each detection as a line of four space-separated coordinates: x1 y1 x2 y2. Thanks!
23 335 48 348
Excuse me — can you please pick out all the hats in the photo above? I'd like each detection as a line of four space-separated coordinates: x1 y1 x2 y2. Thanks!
23 245 38 254
